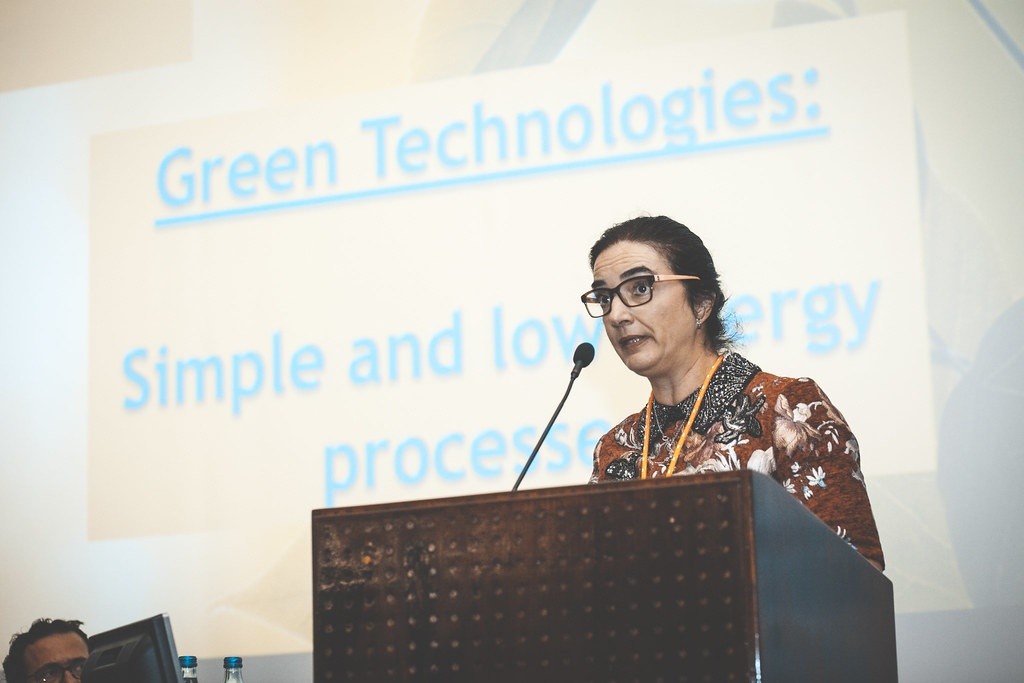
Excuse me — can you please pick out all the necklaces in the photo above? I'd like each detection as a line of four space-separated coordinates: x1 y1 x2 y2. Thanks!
653 391 696 450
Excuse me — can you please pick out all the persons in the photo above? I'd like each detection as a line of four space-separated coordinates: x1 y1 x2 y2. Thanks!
3 618 91 683
588 216 885 575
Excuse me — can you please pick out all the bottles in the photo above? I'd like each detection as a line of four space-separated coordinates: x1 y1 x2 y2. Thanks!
223 656 244 683
178 655 198 683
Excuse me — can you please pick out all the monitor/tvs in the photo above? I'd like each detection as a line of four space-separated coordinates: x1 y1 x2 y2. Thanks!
82 612 184 683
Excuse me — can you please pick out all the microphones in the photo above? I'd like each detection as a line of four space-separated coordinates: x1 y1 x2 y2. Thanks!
511 343 595 492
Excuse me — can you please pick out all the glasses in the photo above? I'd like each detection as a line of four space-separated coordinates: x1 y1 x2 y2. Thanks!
24 659 87 683
581 274 701 319
